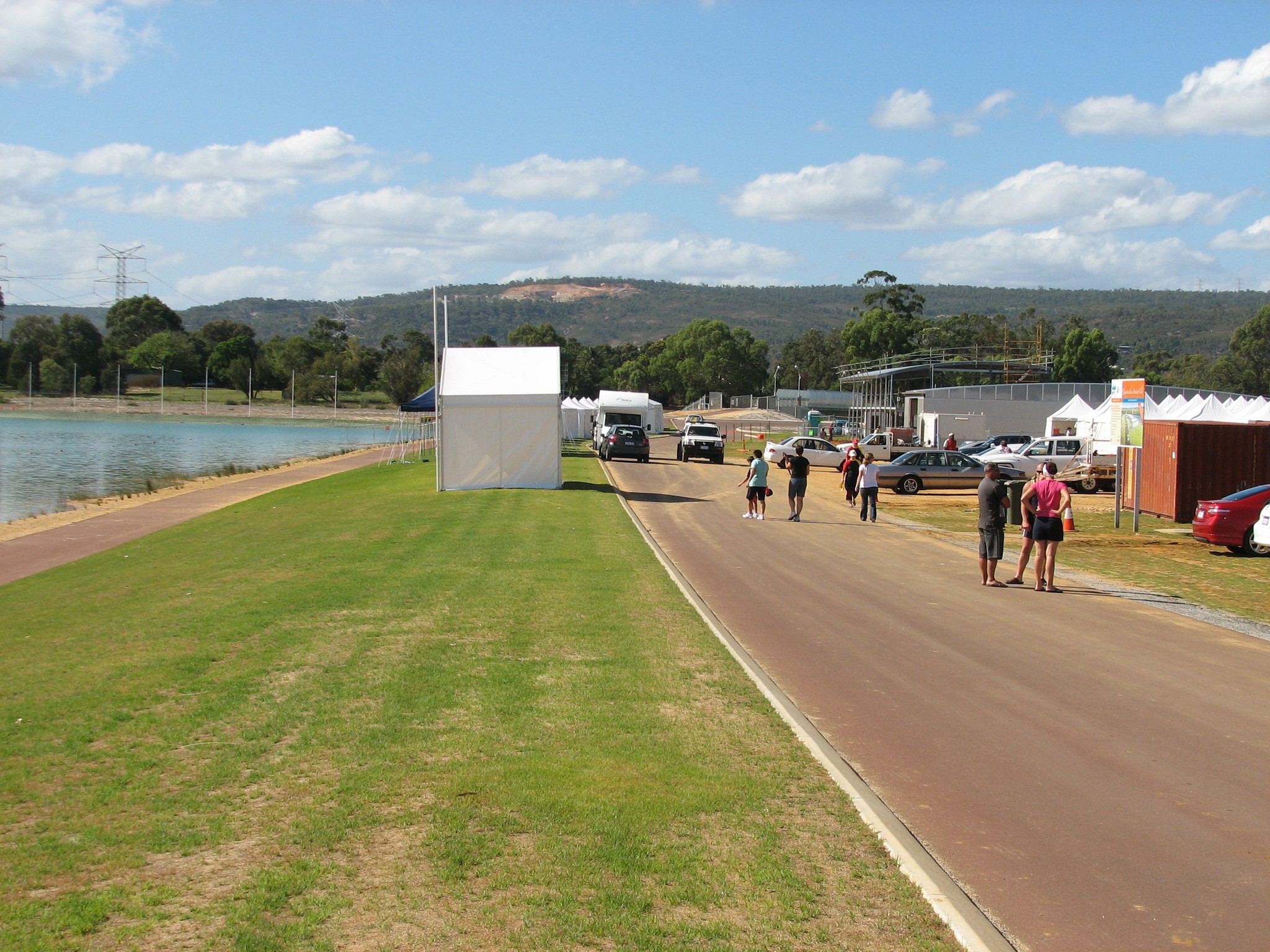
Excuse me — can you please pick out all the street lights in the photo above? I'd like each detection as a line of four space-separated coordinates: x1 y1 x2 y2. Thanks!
794 365 800 397
774 366 780 396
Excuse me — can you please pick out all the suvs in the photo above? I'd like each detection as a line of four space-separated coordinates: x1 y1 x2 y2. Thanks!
677 421 727 464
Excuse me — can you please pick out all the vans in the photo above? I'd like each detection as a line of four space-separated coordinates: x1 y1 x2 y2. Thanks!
684 415 706 425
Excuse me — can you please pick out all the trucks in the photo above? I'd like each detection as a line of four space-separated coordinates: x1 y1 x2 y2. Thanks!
590 390 651 450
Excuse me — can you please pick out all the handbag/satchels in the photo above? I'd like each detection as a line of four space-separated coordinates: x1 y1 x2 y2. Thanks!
764 487 773 496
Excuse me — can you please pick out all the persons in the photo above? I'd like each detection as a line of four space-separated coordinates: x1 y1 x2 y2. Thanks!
1006 462 1047 585
819 427 827 450
738 450 769 520
1021 461 1071 594
1066 427 1072 436
943 433 957 450
829 422 834 441
840 438 880 523
978 462 1011 587
1000 440 1012 453
875 426 881 433
784 445 810 522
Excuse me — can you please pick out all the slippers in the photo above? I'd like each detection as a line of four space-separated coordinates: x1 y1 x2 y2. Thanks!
1040 578 1047 586
1035 586 1045 591
985 581 1008 587
1006 578 1024 584
1045 588 1063 593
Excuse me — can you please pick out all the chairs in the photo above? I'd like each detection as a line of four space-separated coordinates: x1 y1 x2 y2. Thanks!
633 431 638 435
933 453 941 465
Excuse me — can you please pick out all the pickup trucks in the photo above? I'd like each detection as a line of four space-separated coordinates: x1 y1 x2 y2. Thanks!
962 435 1122 494
836 432 938 462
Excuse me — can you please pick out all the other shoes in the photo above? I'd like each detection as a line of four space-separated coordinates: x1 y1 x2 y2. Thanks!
852 498 856 506
849 504 853 507
862 517 866 521
870 518 875 523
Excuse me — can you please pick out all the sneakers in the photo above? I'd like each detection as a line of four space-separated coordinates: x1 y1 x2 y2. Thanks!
757 514 764 520
753 513 758 518
742 512 753 518
789 512 797 520
793 517 799 521
752 509 755 513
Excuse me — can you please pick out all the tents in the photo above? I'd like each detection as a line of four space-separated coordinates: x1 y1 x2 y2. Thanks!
398 348 664 492
1044 394 1270 455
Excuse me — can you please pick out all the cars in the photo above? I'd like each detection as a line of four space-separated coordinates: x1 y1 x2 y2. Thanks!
599 424 650 463
876 449 1030 496
952 433 1075 457
1192 484 1270 557
835 420 865 437
764 436 847 472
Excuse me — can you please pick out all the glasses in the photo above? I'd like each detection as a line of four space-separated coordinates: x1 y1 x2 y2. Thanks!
991 468 999 472
1036 470 1043 474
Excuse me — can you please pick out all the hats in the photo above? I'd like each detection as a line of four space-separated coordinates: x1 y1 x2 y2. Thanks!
849 450 857 457
853 439 858 443
949 433 953 437
